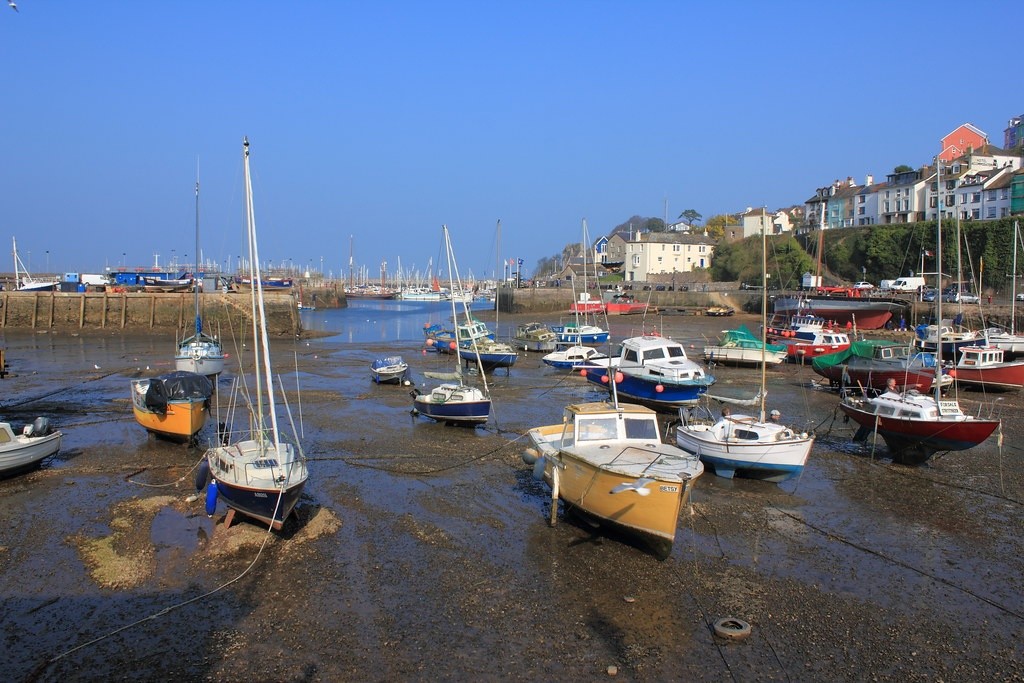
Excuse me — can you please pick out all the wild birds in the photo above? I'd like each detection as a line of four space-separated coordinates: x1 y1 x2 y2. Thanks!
241 344 246 347
367 319 370 322
95 365 101 370
325 320 328 323
307 343 309 346
146 366 149 370
295 334 299 337
137 368 140 371
374 321 376 323
315 356 318 358
134 358 137 361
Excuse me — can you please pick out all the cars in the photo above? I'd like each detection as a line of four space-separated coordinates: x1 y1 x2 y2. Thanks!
923 291 950 303
949 291 980 304
854 282 874 289
1016 291 1024 301
918 284 937 294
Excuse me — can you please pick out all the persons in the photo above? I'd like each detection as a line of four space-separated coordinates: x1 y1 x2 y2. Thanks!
900 318 906 330
885 320 895 330
885 378 923 394
916 346 922 354
767 410 780 423
713 408 729 426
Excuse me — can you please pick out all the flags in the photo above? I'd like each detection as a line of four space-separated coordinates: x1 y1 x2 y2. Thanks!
925 250 933 256
505 258 524 265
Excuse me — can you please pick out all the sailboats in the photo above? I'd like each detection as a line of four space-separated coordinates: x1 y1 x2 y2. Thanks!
421 219 518 369
410 225 492 428
544 273 607 369
759 204 1024 396
12 235 60 291
557 217 611 345
342 234 515 301
205 137 309 532
839 154 1004 467
662 205 818 484
175 155 225 377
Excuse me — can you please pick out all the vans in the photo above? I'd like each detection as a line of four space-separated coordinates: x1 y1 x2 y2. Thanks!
890 276 925 294
81 274 111 287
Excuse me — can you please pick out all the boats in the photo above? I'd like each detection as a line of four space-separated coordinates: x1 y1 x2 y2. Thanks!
143 278 195 292
235 277 293 290
512 321 560 352
702 323 789 368
130 369 213 442
370 356 408 384
706 306 735 317
0 416 64 474
584 286 717 415
521 343 706 559
